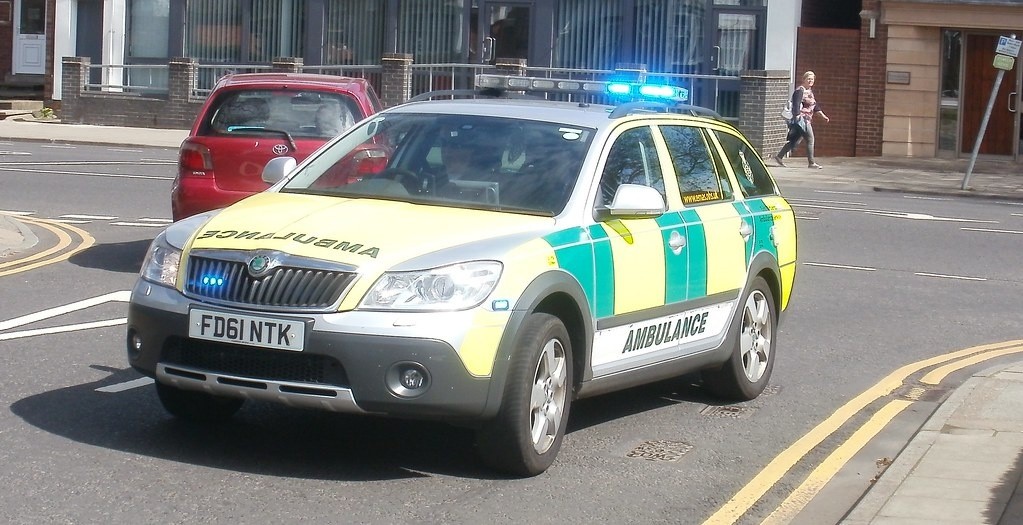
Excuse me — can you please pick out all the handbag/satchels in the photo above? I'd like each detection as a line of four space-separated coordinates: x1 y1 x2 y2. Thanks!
782 86 805 119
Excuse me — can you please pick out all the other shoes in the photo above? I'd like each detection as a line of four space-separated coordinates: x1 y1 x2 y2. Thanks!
775 156 786 166
808 163 822 169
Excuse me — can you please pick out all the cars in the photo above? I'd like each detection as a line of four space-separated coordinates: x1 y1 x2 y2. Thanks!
171 74 394 224
941 90 959 110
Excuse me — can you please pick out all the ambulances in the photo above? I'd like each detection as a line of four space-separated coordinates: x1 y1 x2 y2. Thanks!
126 74 798 479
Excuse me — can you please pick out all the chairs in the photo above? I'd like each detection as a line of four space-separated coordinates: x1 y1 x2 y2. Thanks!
240 97 269 125
315 104 347 132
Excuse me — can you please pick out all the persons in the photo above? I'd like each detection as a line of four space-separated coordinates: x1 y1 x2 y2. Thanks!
417 122 492 198
499 135 573 209
774 71 829 169
